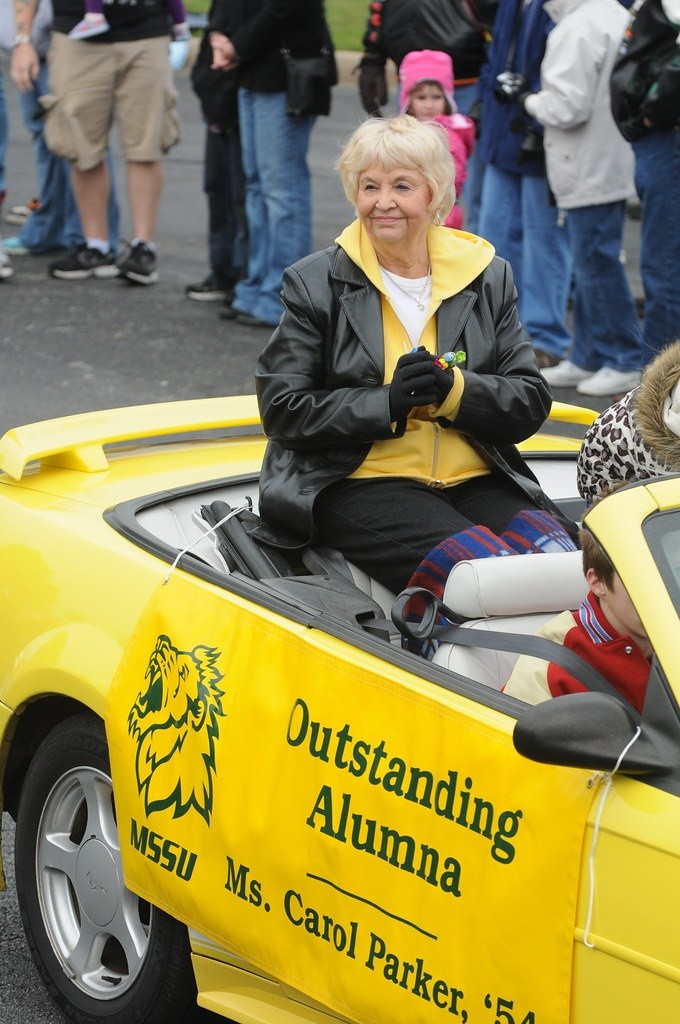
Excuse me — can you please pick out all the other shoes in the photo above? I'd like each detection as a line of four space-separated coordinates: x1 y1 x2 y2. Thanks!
236 312 262 326
218 307 241 319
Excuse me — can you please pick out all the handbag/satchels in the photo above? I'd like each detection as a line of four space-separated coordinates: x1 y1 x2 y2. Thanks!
287 55 331 118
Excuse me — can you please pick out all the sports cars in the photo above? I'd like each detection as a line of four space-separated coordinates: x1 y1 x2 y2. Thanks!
0 381 679 1023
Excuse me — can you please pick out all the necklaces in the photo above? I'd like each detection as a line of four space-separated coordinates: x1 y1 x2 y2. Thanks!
380 263 431 310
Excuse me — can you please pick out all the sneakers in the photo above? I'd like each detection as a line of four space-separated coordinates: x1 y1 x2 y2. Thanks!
1 235 32 256
578 365 644 397
49 241 119 281
116 237 160 284
185 271 225 304
537 361 597 386
9 198 40 225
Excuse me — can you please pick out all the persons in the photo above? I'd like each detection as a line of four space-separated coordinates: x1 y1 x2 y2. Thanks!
499 479 655 715
576 336 680 506
245 116 581 662
187 0 340 328
0 0 195 287
351 0 679 397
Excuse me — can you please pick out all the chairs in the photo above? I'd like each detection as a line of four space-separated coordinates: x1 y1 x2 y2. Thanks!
433 547 590 692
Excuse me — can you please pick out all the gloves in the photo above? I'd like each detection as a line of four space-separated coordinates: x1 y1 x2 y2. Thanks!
389 346 437 420
351 55 389 119
409 347 454 407
